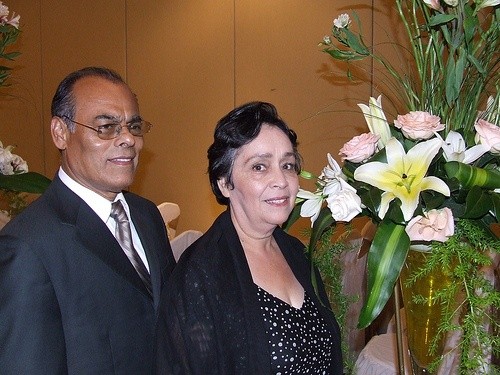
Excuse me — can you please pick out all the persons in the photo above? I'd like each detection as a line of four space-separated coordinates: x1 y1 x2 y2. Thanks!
154 101 344 375
0 68 176 375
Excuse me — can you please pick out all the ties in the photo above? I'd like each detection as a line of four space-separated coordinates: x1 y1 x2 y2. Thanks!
109 199 153 305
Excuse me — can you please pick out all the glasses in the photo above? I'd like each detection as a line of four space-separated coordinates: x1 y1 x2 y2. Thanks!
61 115 152 140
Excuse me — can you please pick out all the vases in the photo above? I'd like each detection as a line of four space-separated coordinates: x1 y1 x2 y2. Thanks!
398 245 500 375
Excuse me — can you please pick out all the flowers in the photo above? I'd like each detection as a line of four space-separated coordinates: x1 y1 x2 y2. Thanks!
301 0 500 375
0 0 51 211
